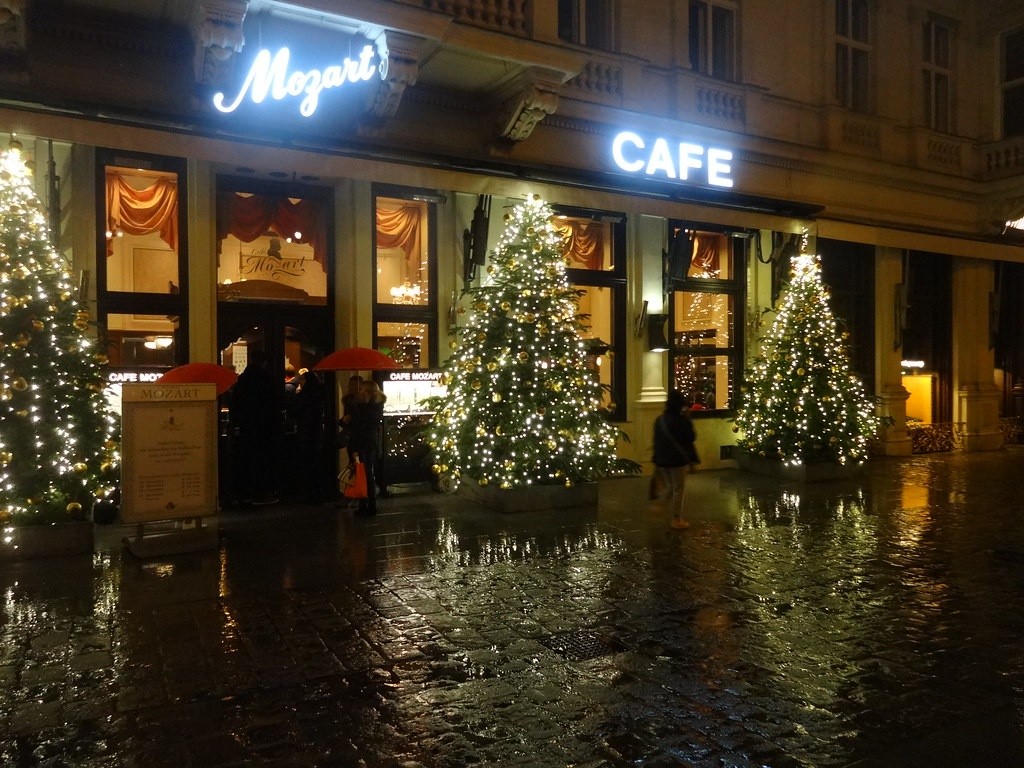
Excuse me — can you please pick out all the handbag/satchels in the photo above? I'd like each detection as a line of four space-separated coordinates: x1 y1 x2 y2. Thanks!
337 457 368 498
650 469 668 492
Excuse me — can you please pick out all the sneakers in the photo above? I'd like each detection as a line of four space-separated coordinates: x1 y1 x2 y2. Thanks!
671 518 691 529
252 494 281 505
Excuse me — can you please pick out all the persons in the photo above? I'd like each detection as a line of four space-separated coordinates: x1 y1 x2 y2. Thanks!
649 390 700 528
221 346 326 503
337 380 386 517
690 391 716 410
337 375 364 434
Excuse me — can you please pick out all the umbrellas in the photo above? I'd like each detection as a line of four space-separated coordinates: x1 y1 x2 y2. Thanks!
313 346 400 398
152 362 240 397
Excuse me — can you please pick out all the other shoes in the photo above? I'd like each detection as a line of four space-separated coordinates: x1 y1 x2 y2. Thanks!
353 503 377 518
